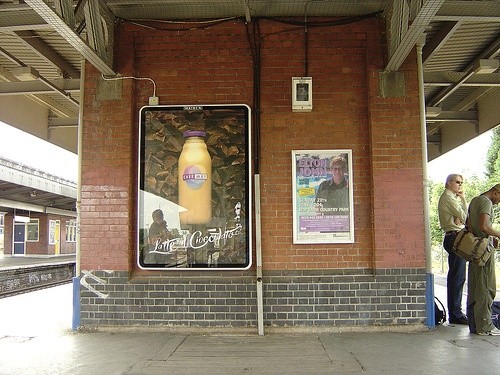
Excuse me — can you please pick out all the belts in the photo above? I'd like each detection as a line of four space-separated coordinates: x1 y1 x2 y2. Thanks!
446 230 458 234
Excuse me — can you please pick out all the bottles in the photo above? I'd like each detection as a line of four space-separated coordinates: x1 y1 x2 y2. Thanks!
177 131 212 224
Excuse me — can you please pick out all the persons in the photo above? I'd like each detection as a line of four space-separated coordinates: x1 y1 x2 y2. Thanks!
464 183 500 336
314 157 350 233
438 174 468 325
140 208 233 268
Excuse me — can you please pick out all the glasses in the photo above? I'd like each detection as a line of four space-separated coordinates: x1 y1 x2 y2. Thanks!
452 180 463 184
333 168 344 171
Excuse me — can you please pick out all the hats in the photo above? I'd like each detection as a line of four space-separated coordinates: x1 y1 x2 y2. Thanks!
493 183 500 191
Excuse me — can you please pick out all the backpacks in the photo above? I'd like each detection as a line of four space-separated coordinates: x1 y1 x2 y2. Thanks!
435 296 446 324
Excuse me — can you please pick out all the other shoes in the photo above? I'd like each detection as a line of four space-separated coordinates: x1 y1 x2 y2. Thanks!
478 327 500 336
449 316 468 324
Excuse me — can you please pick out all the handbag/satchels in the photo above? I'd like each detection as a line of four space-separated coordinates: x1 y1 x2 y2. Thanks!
453 227 493 266
